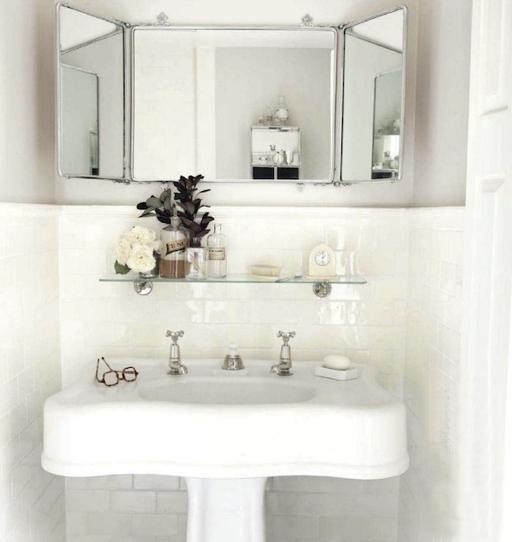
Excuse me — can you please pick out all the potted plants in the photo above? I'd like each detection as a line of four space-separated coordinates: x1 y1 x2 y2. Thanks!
135 174 214 279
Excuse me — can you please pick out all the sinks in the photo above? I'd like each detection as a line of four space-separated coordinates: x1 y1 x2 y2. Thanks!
41 356 411 480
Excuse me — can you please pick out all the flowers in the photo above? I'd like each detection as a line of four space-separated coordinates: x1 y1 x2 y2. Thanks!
113 225 163 272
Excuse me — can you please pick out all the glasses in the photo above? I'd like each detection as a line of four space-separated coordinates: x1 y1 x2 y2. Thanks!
96 357 138 386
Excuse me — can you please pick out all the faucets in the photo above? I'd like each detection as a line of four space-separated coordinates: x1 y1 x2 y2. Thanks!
269 330 295 375
222 344 245 369
164 330 189 376
